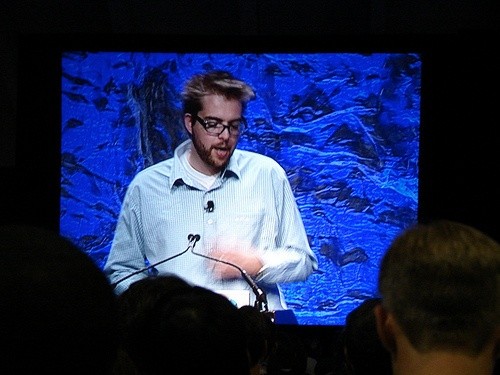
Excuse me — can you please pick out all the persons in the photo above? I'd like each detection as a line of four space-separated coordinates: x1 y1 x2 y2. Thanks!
101 74 318 319
0 216 500 375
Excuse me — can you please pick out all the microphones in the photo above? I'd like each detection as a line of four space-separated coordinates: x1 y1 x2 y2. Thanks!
112 233 268 312
208 201 214 213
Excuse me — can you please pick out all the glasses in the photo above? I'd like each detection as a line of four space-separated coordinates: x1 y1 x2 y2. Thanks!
192 114 248 136
